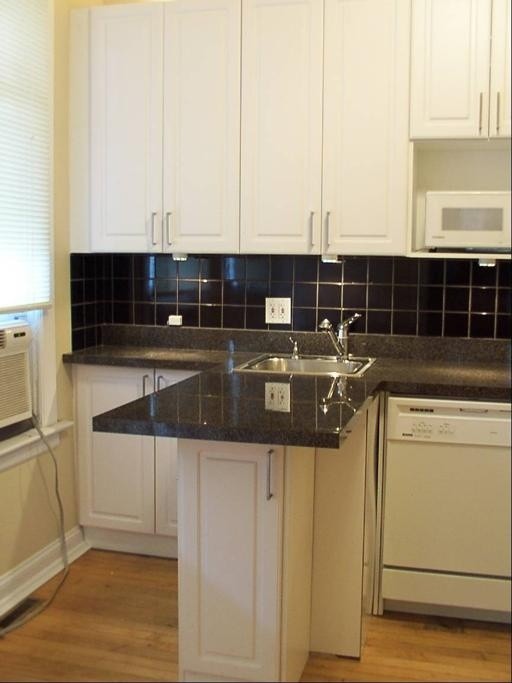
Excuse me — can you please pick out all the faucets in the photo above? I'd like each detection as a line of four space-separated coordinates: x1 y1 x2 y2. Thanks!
318 377 358 415
318 312 363 357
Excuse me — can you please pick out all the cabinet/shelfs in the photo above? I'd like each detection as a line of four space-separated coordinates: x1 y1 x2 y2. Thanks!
411 0 510 139
175 437 315 681
69 0 410 257
71 360 210 558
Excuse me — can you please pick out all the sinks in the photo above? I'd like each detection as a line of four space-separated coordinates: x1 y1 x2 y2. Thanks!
231 352 377 377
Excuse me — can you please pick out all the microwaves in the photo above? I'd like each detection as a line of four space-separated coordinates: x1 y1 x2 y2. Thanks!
423 189 511 251
